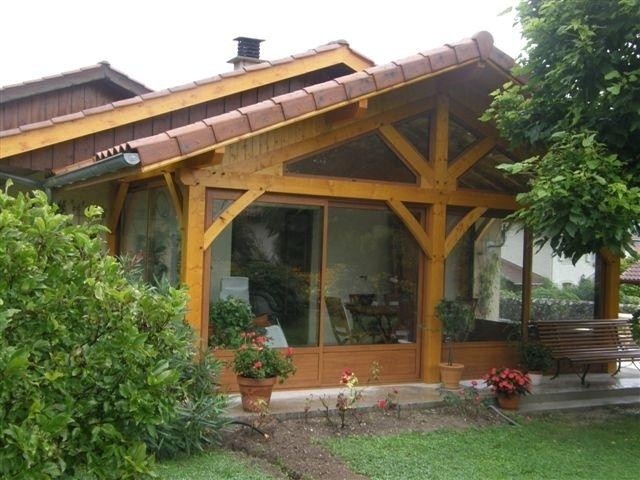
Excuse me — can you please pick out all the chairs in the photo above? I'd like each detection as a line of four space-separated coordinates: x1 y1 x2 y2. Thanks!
220 276 289 348
323 292 477 345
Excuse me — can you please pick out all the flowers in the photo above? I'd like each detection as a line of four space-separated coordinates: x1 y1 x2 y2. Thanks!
230 331 298 385
480 363 534 396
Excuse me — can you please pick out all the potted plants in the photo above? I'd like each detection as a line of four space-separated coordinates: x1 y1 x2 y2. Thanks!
520 342 555 387
436 300 476 390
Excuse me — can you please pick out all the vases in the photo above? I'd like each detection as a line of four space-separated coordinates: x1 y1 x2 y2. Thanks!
237 374 275 411
497 392 519 409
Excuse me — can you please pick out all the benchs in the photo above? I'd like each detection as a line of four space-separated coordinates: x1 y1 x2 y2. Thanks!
538 319 640 385
